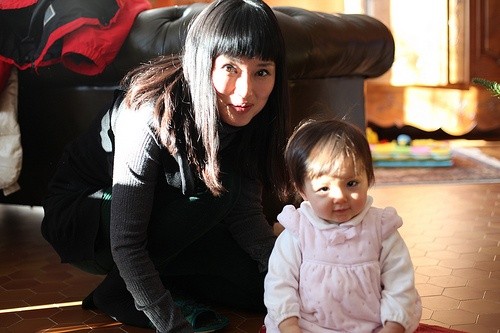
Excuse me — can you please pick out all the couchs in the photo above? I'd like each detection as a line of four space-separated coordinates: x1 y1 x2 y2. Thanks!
13 1 399 206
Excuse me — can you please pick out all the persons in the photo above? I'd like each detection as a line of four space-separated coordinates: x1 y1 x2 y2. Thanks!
263 119 421 333
41 0 288 332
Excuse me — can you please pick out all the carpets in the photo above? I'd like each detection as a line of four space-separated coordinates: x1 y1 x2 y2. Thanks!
370 141 500 188
